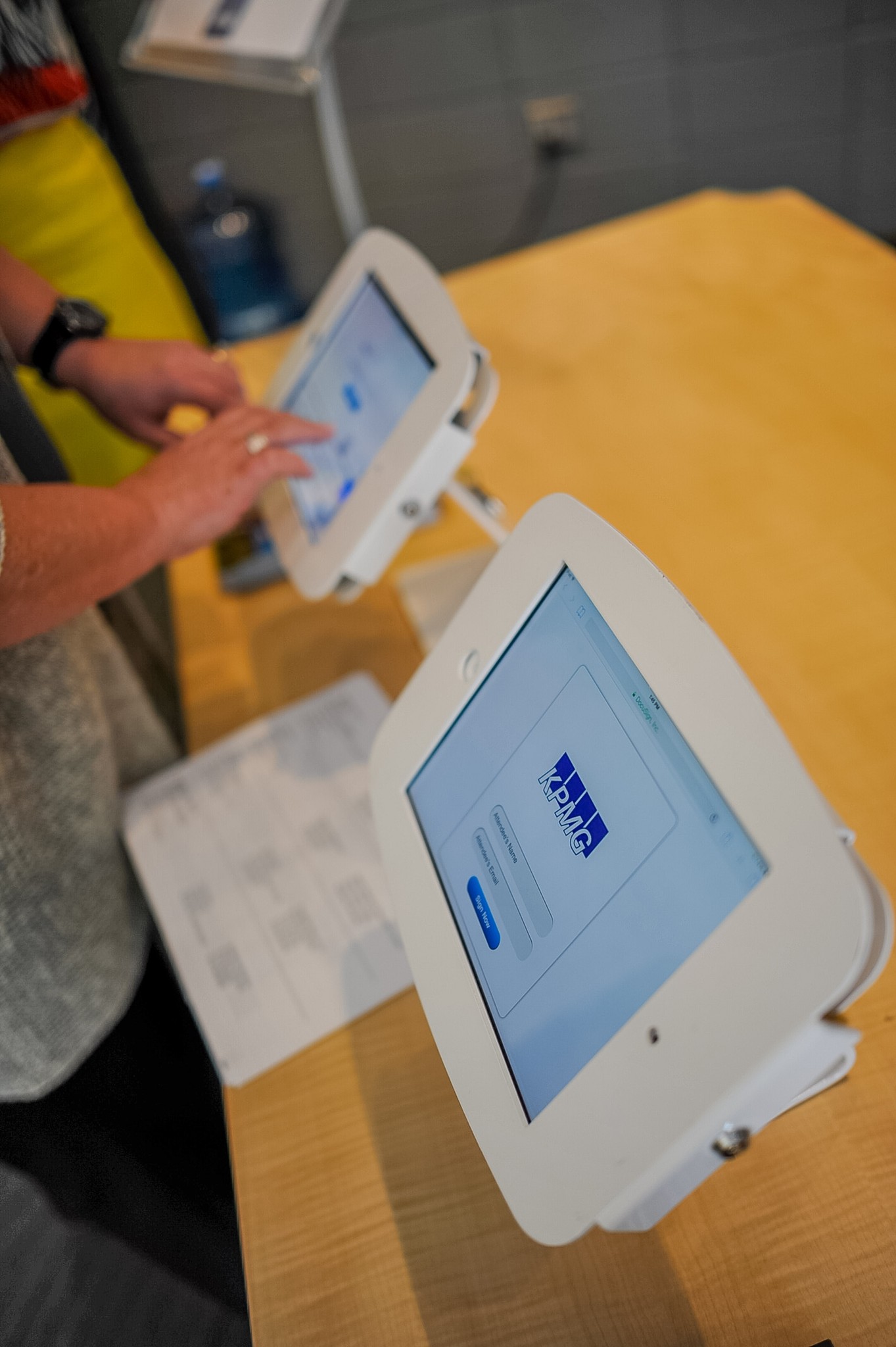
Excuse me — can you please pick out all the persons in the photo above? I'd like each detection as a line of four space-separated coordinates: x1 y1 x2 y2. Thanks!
1 239 334 1316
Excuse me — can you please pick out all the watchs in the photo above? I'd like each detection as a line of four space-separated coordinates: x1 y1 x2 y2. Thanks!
31 298 107 389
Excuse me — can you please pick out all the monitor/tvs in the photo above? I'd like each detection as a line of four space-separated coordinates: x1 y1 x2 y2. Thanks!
258 226 475 600
366 494 877 1244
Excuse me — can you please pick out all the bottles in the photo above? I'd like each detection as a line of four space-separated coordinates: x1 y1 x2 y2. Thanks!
180 157 294 347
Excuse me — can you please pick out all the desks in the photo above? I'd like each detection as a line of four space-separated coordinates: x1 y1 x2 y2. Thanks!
161 184 896 1347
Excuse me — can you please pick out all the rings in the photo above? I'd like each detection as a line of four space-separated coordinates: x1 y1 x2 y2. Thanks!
247 432 270 453
